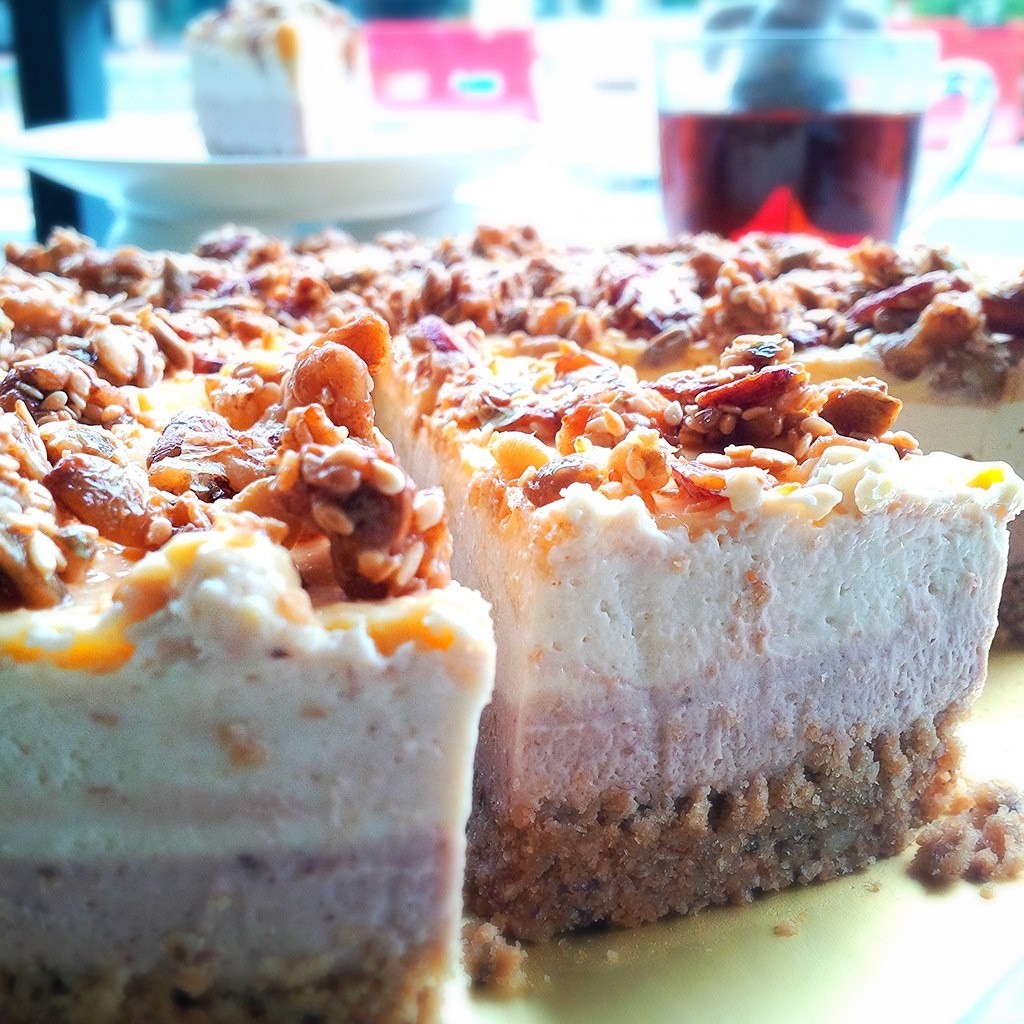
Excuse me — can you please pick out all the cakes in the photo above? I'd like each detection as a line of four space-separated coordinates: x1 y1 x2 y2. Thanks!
185 0 377 159
0 223 1024 1024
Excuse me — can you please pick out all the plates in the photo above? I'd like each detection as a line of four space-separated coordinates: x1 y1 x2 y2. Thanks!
6 112 466 223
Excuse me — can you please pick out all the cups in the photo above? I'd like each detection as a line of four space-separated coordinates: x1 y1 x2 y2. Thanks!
649 31 1000 252
